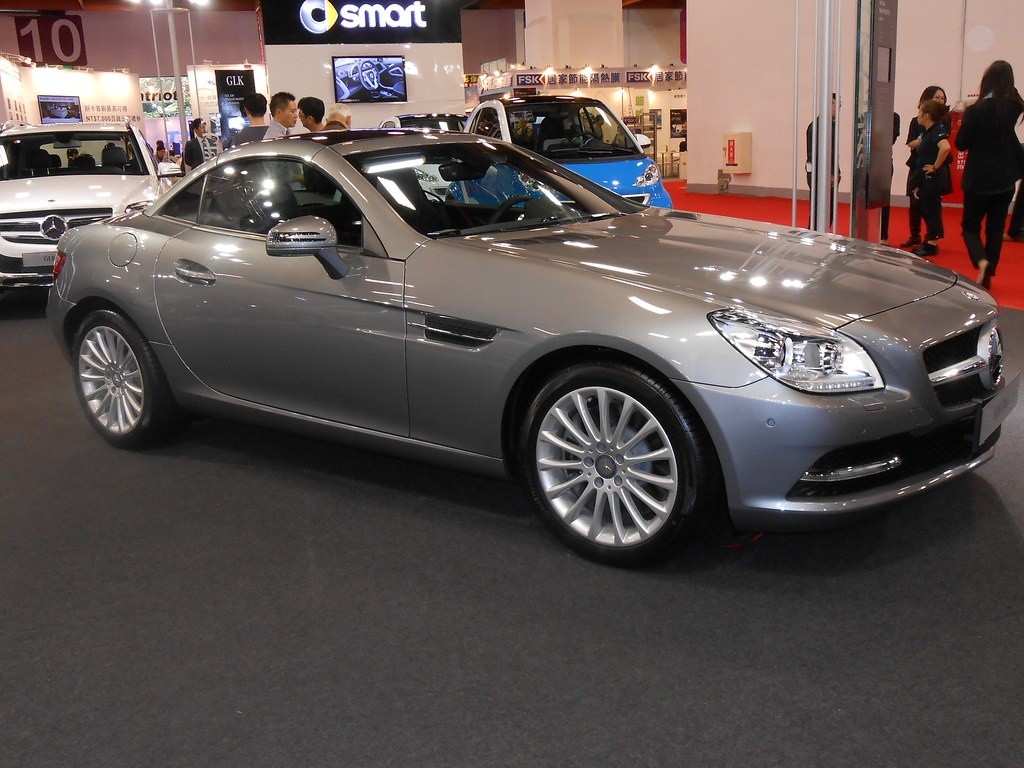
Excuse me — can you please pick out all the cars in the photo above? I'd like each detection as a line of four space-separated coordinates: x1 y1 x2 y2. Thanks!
48 130 1023 567
377 113 468 205
444 96 673 209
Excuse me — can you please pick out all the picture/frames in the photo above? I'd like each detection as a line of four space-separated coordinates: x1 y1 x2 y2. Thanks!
37 95 83 125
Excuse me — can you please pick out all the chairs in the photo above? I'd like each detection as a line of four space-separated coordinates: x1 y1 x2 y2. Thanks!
25 150 51 177
101 147 128 174
49 154 62 170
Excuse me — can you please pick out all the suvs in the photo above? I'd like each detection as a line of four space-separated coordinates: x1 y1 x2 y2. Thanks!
0 120 183 318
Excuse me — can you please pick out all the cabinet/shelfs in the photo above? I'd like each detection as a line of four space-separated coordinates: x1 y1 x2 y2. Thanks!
624 113 658 162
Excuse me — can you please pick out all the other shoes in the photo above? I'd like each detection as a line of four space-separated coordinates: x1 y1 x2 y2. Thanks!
1002 234 1024 242
977 263 991 289
900 237 920 247
911 243 937 256
881 239 893 247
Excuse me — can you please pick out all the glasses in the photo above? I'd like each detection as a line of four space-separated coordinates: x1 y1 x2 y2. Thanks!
933 96 946 102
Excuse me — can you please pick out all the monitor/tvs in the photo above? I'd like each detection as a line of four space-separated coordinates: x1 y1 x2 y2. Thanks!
332 56 407 103
37 95 83 124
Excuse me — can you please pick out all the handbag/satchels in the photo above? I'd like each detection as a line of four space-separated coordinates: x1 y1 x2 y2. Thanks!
932 166 953 195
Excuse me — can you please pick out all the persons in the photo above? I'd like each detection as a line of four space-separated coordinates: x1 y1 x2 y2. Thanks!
954 61 1024 290
805 93 841 231
230 93 270 148
611 121 627 146
593 114 605 141
297 96 325 132
185 118 217 172
262 92 299 142
154 140 178 165
899 86 954 256
861 110 900 246
679 137 687 152
321 103 352 131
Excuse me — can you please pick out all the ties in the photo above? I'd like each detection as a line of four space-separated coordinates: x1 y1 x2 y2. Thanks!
286 131 290 140
202 140 209 161
